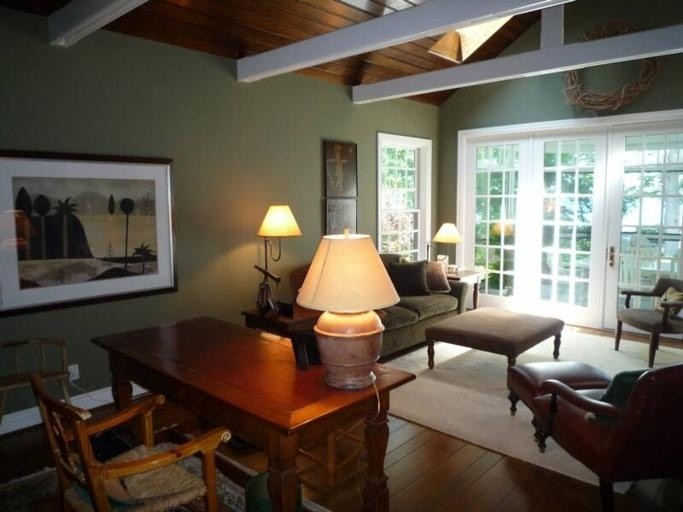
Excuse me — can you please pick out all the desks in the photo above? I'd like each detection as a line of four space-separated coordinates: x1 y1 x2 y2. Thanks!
90 315 416 512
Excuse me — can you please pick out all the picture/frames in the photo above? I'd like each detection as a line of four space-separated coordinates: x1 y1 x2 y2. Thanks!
322 140 359 198
0 149 178 319
324 199 358 235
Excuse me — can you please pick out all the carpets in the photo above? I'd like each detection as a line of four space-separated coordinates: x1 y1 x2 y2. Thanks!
0 424 331 512
387 329 683 495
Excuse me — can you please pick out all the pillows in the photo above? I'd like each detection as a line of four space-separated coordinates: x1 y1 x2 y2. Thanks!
402 258 452 294
588 369 651 430
655 286 683 318
388 261 431 296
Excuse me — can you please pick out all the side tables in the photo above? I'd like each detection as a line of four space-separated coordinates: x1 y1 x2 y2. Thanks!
446 270 482 309
241 302 291 332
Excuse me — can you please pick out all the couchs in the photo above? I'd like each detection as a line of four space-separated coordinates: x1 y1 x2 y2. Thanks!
290 254 469 358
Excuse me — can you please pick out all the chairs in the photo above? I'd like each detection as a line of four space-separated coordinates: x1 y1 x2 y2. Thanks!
507 360 683 512
615 278 683 368
29 371 233 512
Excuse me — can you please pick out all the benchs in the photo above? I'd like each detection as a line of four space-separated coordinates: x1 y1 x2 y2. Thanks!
0 338 71 425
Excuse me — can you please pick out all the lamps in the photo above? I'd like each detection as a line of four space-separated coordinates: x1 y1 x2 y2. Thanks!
296 228 400 389
427 223 464 262
253 205 303 312
